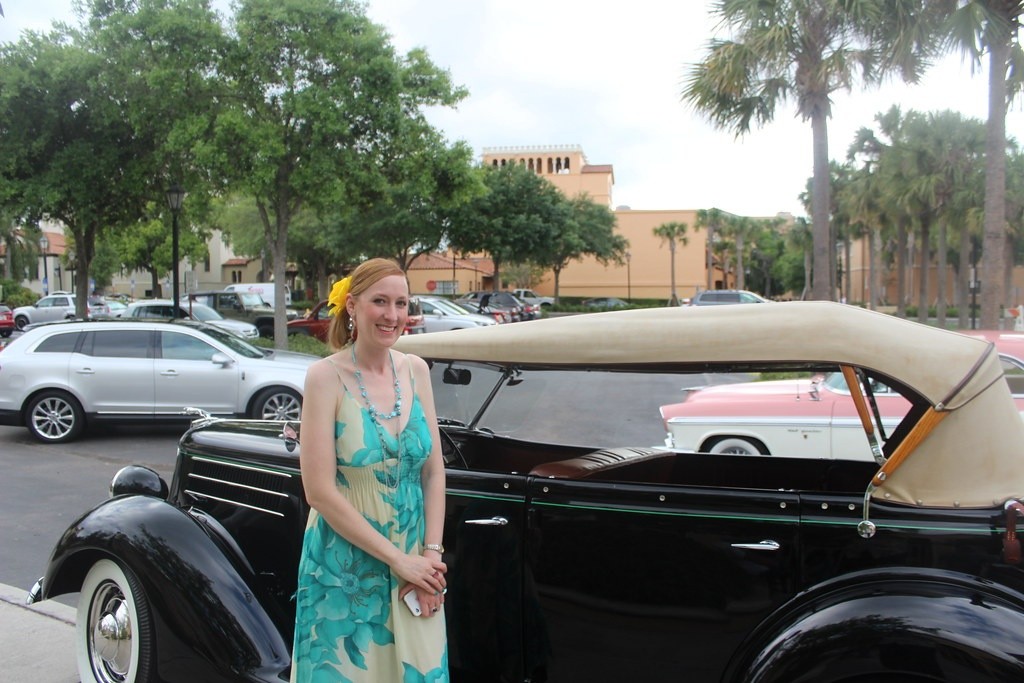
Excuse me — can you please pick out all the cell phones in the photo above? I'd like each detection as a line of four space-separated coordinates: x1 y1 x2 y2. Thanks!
403 588 447 617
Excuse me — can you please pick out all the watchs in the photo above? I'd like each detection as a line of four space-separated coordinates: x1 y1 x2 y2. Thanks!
422 545 444 553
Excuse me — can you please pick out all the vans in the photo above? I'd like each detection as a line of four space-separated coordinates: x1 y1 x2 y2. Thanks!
224 283 293 308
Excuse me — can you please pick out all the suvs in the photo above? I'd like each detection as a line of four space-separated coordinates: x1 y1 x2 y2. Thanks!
683 289 771 305
13 291 109 331
188 291 298 336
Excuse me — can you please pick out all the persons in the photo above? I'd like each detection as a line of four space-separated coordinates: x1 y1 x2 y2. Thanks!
290 258 450 683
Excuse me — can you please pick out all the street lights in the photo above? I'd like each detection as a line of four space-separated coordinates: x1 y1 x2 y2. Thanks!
624 253 632 303
68 250 76 293
164 183 186 319
39 235 50 296
473 260 479 291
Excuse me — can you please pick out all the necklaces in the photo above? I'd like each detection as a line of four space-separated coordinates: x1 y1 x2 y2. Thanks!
352 343 403 503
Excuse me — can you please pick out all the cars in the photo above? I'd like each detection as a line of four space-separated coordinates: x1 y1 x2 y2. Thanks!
461 289 555 322
582 297 628 307
287 294 426 343
0 303 16 336
0 319 325 444
409 294 512 332
110 294 138 303
122 300 260 344
100 297 127 319
25 294 1024 683
658 328 1024 461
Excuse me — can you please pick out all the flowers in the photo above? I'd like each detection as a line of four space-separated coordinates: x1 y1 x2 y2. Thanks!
325 274 353 316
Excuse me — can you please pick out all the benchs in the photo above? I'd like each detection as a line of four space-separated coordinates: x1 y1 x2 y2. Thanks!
529 442 670 479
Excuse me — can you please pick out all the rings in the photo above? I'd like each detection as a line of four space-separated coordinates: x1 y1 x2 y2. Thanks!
431 608 437 612
432 570 439 578
